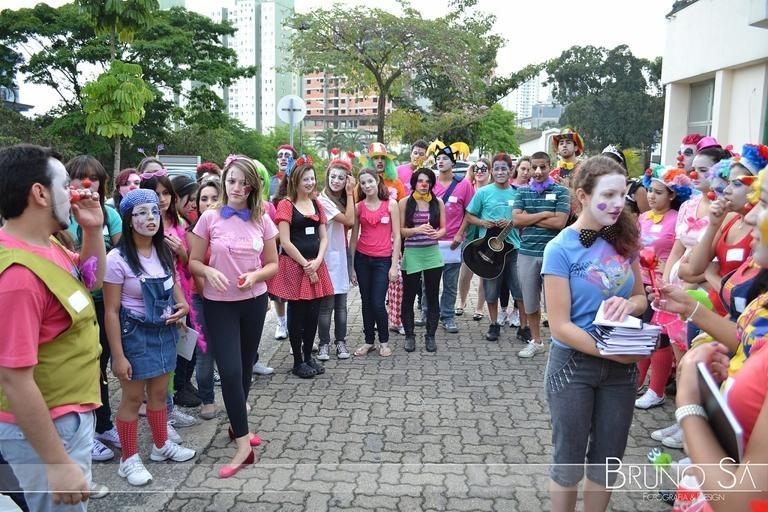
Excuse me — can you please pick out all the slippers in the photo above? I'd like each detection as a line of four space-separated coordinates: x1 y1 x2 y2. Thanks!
306 359 325 374
472 312 484 320
292 362 317 378
454 308 463 315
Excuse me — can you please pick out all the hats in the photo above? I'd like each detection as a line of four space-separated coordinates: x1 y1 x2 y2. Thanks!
551 127 583 156
119 188 160 216
738 143 767 176
359 142 397 165
425 141 469 164
170 175 199 193
642 165 690 202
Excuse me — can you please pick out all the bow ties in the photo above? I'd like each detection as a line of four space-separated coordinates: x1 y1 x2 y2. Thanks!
578 225 616 248
557 160 575 171
413 191 432 202
645 212 663 224
220 206 252 222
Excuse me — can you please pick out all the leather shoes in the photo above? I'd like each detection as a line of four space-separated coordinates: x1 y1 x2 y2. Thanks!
426 335 436 352
404 336 415 352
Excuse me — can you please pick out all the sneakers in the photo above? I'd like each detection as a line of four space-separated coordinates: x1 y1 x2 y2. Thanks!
166 381 217 445
213 368 221 386
633 381 675 409
289 340 349 360
93 425 122 449
485 311 548 358
88 482 109 499
442 320 457 333
669 458 694 475
150 440 196 463
274 322 286 339
413 317 426 326
650 423 683 442
118 453 152 486
252 362 273 375
662 428 686 449
90 439 114 461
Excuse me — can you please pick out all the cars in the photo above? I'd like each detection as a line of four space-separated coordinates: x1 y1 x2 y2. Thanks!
510 158 521 165
160 167 201 187
431 160 472 178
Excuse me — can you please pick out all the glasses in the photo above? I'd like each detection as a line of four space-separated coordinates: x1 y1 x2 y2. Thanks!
130 210 161 218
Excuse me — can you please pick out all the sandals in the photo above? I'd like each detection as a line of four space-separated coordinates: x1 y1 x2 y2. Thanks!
378 343 392 357
352 345 376 356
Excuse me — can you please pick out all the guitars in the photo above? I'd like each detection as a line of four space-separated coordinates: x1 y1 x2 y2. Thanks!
461 221 515 279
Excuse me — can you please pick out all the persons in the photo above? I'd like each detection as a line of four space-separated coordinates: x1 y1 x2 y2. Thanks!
0 128 768 512
